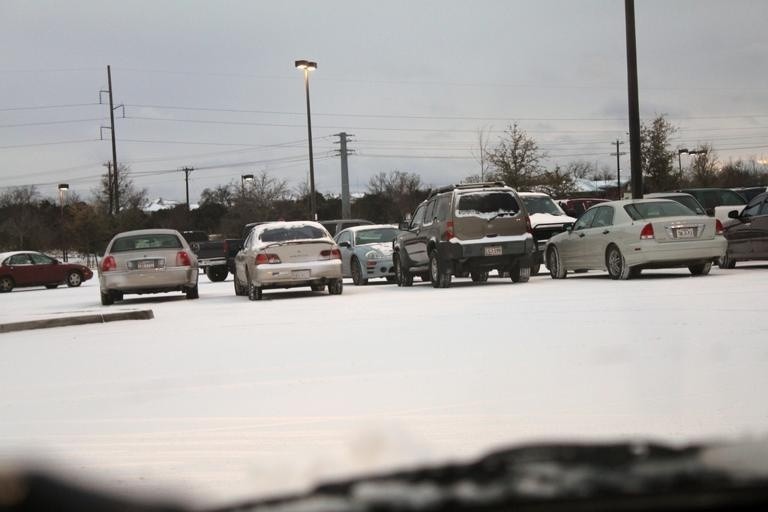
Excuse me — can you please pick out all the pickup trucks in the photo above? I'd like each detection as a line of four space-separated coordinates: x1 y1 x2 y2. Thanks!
191 220 278 283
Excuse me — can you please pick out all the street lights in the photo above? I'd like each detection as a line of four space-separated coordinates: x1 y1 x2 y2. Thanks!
56 182 71 263
293 58 321 217
242 174 254 198
677 148 688 179
689 151 708 174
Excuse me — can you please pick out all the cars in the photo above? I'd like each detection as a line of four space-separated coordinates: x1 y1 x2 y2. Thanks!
228 219 345 301
97 226 202 306
0 249 94 294
